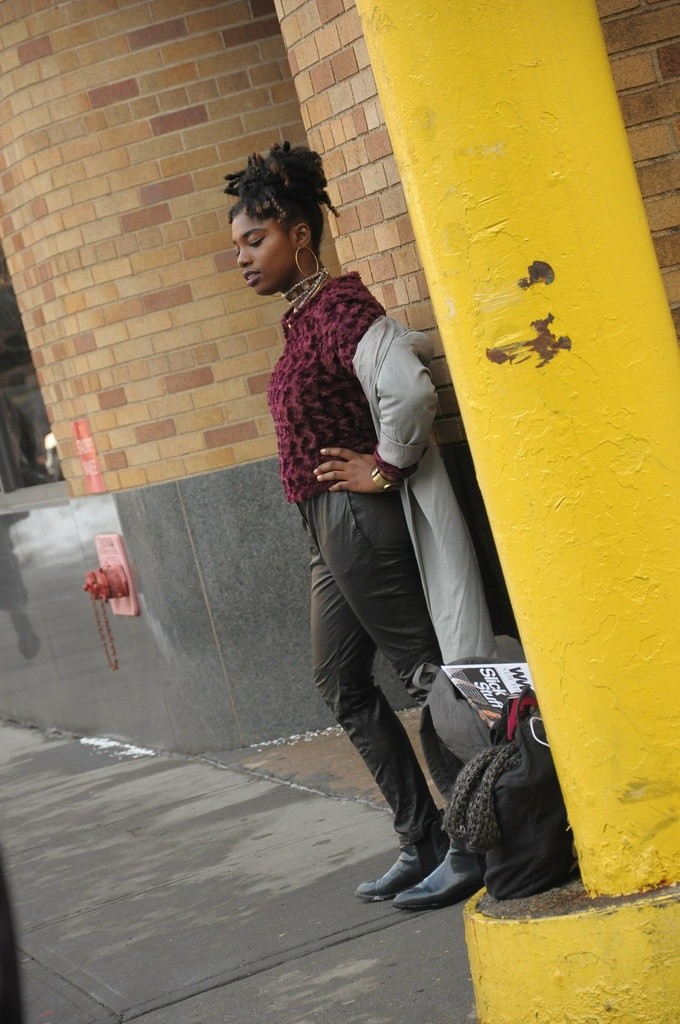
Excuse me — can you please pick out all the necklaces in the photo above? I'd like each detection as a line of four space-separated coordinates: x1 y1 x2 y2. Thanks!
282 269 329 309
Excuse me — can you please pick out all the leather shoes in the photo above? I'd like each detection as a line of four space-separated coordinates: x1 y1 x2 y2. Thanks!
392 836 488 910
355 820 451 901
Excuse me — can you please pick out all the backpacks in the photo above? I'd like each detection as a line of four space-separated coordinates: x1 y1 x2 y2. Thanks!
412 657 581 901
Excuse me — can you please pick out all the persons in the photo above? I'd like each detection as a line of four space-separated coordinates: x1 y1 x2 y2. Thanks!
230 144 487 911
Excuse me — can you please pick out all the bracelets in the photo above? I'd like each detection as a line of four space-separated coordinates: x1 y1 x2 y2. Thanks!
371 464 403 490
